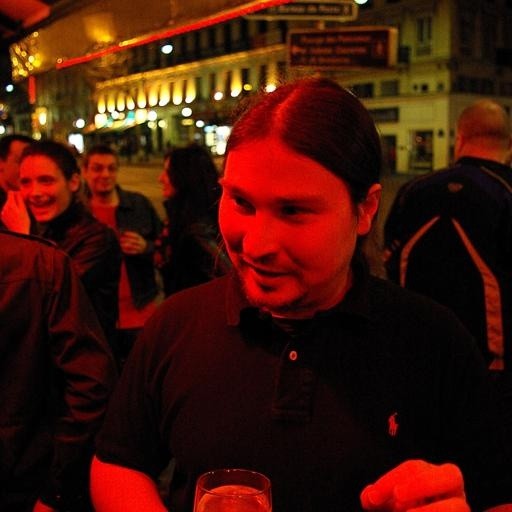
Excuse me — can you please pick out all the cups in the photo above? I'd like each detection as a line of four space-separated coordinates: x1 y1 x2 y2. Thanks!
193 467 273 512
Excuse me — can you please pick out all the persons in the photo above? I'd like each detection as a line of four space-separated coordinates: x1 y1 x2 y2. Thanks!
154 140 233 300
87 74 512 512
0 134 38 231
0 139 120 372
385 97 512 413
78 145 166 357
0 183 116 512
